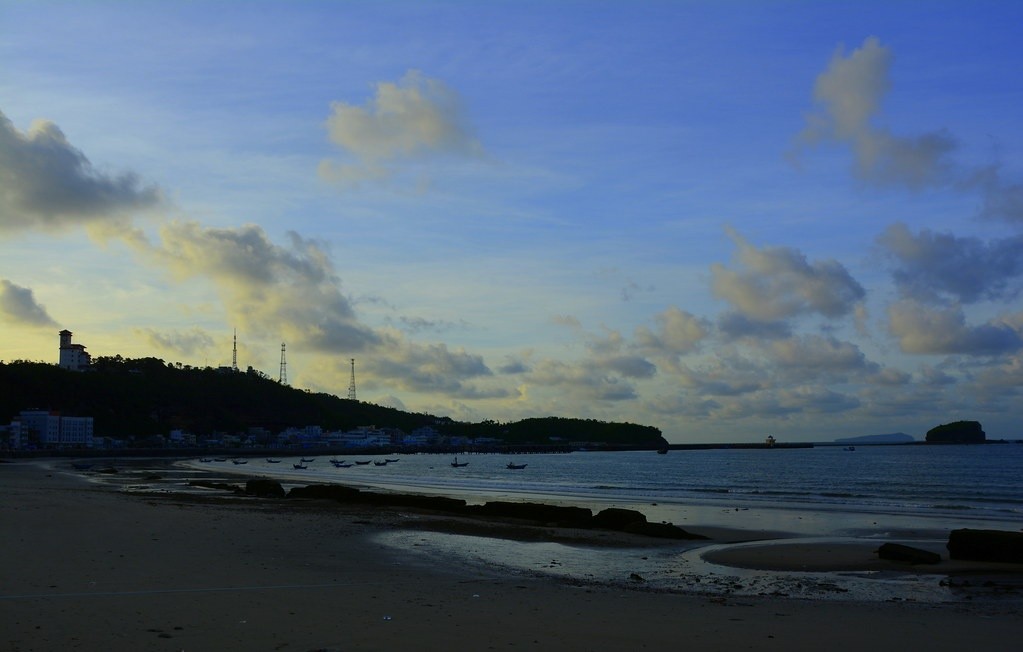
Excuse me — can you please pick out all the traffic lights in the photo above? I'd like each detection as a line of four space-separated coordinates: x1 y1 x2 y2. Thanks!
231 459 249 465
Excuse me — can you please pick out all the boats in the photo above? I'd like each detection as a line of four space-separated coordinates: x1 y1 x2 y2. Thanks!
266 457 282 463
384 458 400 462
214 457 226 461
300 456 315 462
355 460 372 465
373 460 388 465
843 446 855 451
200 458 212 462
507 461 528 469
330 458 346 463
334 462 354 468
292 462 308 470
450 456 470 467
